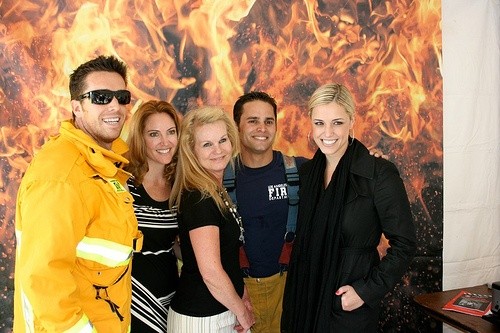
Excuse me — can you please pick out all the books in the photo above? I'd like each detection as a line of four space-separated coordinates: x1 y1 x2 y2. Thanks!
441 290 492 317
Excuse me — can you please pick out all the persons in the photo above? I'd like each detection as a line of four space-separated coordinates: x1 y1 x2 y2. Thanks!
12 55 141 333
279 84 414 333
114 100 181 333
167 106 257 333
219 90 310 333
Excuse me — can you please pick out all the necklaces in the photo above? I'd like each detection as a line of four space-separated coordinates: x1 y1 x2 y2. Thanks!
219 188 244 241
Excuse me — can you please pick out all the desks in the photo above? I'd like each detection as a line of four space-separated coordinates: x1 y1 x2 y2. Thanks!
412 283 500 333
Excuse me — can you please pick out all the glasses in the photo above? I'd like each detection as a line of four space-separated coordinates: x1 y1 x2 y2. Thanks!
79 88 131 104
